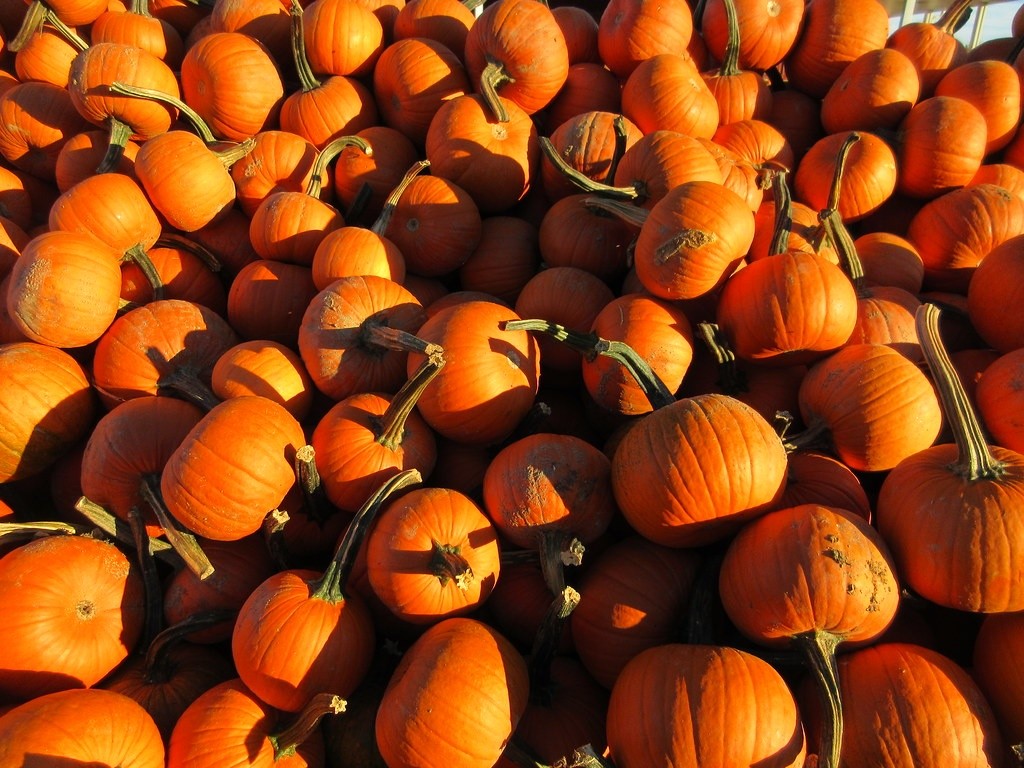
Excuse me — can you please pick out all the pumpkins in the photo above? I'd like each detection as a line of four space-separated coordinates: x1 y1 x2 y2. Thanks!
0 0 1024 768
